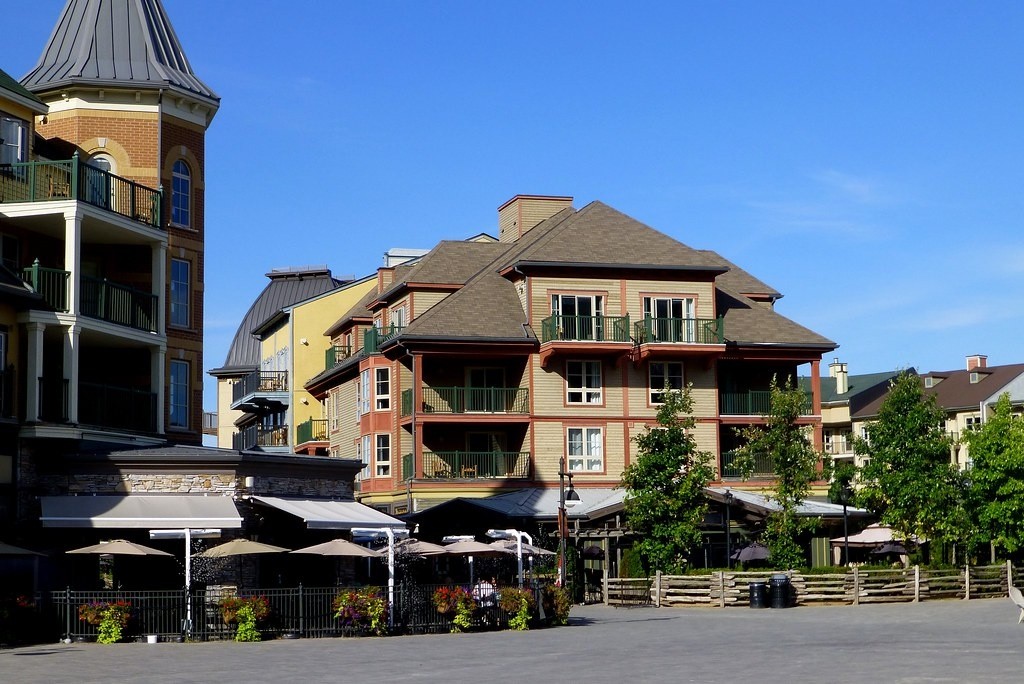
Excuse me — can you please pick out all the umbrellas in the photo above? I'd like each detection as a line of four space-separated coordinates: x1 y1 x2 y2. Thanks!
288 538 385 588
489 539 557 585
442 534 502 585
63 538 175 591
192 537 291 589
372 537 443 587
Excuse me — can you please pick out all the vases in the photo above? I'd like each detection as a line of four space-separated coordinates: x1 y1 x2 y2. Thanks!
86 613 100 624
223 611 236 624
437 602 449 613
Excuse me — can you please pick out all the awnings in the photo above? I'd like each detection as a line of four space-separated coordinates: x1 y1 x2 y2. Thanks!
251 494 408 535
33 487 243 533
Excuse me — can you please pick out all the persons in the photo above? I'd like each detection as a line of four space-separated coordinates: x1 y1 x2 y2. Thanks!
470 573 502 608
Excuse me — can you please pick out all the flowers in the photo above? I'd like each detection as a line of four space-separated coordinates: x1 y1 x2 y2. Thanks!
78 601 132 645
546 580 573 625
333 585 392 638
497 587 533 631
217 595 271 642
432 586 477 634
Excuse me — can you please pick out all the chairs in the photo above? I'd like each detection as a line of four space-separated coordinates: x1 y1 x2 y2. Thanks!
432 460 478 479
48 178 70 198
261 376 284 391
422 401 434 413
275 428 287 446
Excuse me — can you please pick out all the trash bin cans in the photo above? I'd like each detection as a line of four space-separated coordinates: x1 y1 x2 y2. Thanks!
748 581 767 608
768 578 791 608
771 574 787 583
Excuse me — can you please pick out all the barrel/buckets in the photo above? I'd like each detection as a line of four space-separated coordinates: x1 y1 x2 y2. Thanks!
148 635 157 643
748 581 767 609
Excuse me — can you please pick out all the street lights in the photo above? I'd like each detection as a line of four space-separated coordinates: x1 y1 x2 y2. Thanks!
722 490 733 570
555 456 584 588
839 485 851 566
